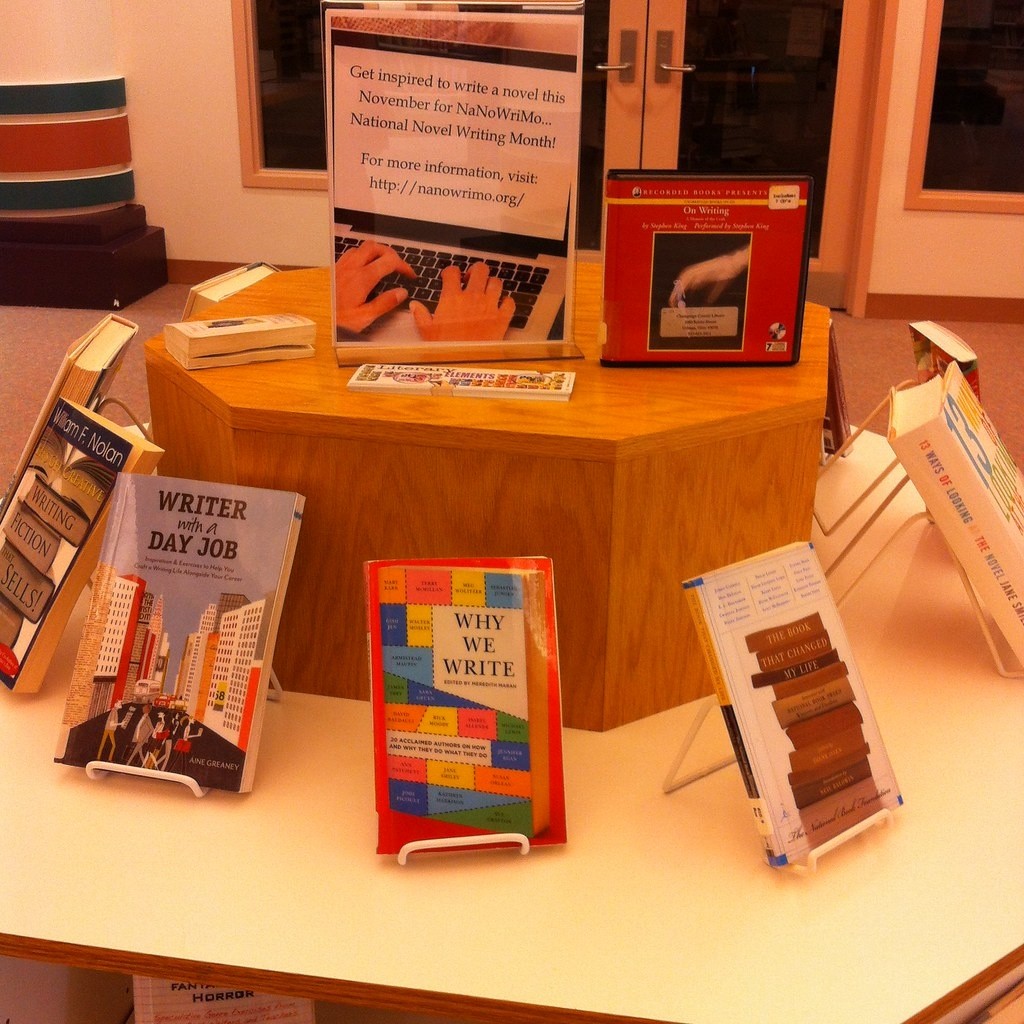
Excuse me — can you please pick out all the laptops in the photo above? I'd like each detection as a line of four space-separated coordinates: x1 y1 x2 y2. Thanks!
329 28 575 344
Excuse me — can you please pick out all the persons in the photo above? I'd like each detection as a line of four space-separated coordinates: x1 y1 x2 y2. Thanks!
663 249 751 308
331 240 519 345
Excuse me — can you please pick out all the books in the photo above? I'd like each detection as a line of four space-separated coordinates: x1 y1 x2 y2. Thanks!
1 166 1024 1023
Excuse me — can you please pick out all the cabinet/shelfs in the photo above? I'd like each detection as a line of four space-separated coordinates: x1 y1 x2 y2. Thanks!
1 270 1023 1024
